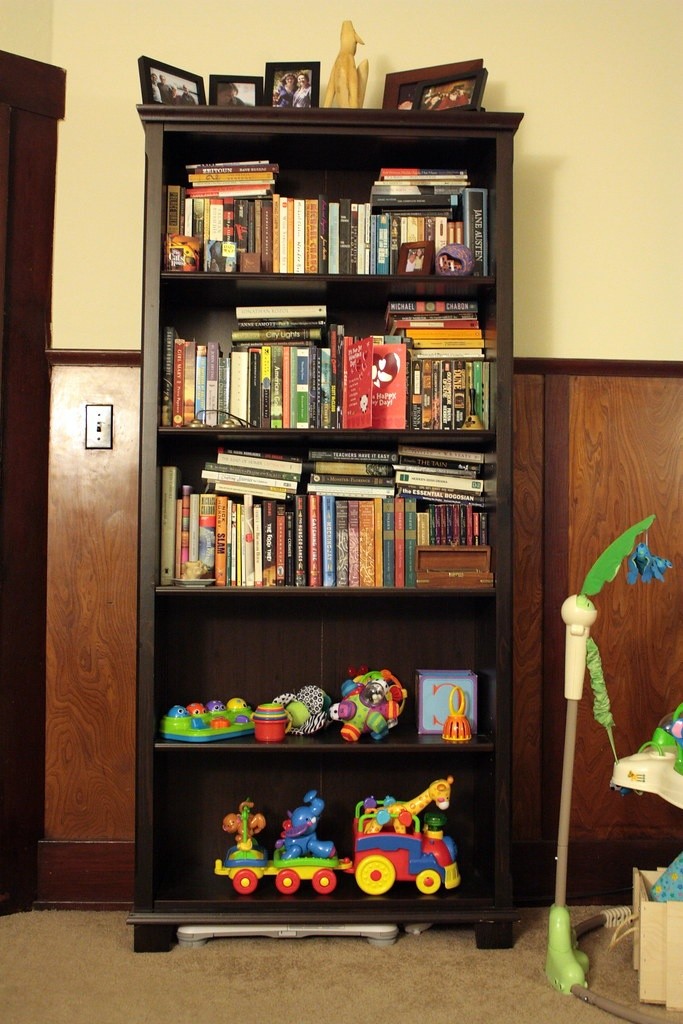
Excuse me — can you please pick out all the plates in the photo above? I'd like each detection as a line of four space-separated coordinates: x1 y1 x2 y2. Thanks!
171 578 216 587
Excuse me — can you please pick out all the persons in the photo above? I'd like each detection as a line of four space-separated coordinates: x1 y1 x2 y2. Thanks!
276 73 298 108
181 85 196 105
406 252 416 272
293 73 311 108
415 248 424 272
151 73 174 105
217 82 244 106
423 87 471 110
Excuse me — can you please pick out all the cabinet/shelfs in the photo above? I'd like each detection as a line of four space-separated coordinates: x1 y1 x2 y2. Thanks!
126 110 532 955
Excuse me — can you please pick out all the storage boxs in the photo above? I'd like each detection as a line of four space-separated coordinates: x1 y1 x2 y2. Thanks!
415 669 478 737
630 866 682 1012
415 545 495 587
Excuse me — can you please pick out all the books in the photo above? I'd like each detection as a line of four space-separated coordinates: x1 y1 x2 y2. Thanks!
159 300 490 429
165 160 488 276
159 444 490 588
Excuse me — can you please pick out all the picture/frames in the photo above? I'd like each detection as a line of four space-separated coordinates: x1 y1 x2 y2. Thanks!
412 68 489 112
265 61 322 108
382 59 484 110
207 75 266 104
396 241 435 275
137 55 207 105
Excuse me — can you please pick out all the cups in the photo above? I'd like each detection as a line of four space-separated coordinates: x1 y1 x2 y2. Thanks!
251 702 291 743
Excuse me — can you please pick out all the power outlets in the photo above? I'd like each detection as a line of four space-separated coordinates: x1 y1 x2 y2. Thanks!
85 403 114 450
